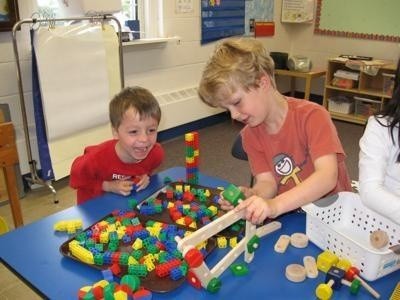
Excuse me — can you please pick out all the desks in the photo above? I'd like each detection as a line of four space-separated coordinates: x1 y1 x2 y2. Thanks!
0 166 400 300
273 69 326 101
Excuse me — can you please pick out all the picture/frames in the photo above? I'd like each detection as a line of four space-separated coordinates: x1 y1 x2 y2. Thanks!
0 0 21 33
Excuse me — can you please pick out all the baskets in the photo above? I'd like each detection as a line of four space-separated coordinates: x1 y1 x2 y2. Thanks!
300 191 400 281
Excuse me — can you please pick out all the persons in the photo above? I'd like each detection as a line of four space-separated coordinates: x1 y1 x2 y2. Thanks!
196 36 352 225
357 59 399 225
69 85 166 204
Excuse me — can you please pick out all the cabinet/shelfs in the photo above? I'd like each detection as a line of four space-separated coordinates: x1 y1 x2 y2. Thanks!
322 59 397 125
